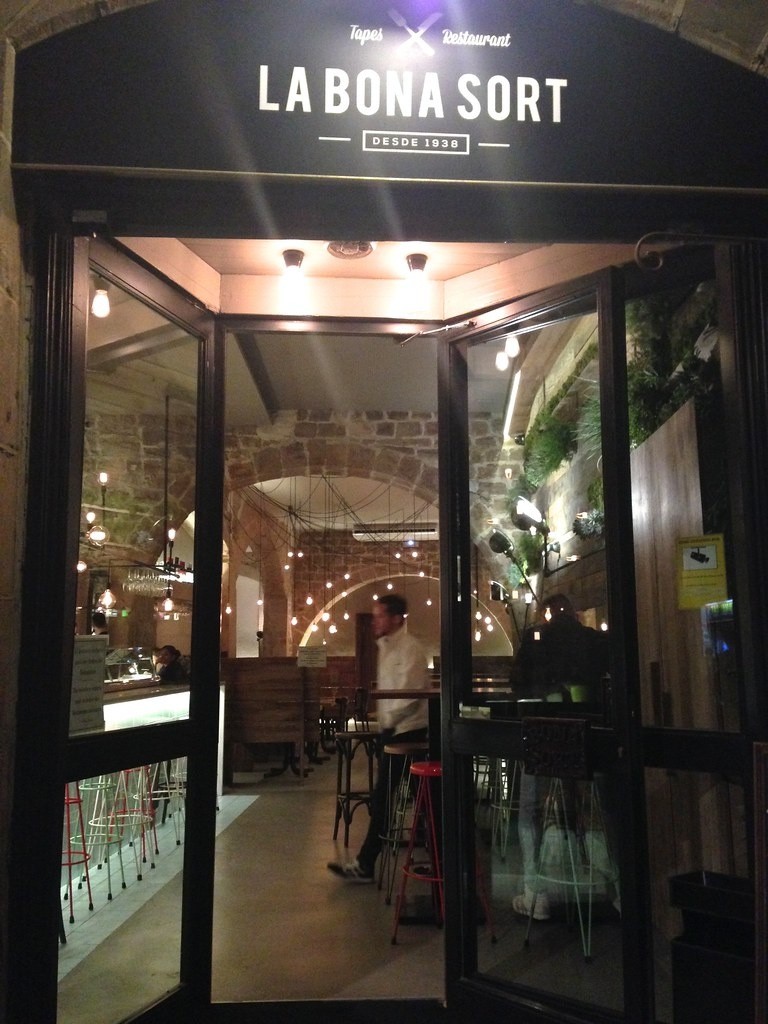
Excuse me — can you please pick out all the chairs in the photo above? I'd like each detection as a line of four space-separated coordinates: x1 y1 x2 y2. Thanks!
343 686 369 732
319 695 349 753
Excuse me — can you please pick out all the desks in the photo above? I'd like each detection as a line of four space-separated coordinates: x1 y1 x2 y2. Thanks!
417 671 517 690
364 684 522 896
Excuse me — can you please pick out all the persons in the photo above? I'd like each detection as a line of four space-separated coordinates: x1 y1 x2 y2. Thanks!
157 645 186 685
510 593 622 920
90 613 109 646
328 594 431 883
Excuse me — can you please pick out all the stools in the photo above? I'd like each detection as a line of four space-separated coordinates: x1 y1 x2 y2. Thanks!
380 711 622 965
333 731 383 847
58 751 195 952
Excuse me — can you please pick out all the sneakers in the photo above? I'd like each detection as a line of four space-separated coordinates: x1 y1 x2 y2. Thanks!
512 884 551 920
414 864 434 879
327 861 375 883
612 884 622 918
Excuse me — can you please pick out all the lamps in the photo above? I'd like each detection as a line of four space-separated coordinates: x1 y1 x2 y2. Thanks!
504 337 521 358
512 496 552 577
495 351 509 371
489 528 546 615
77 472 187 622
